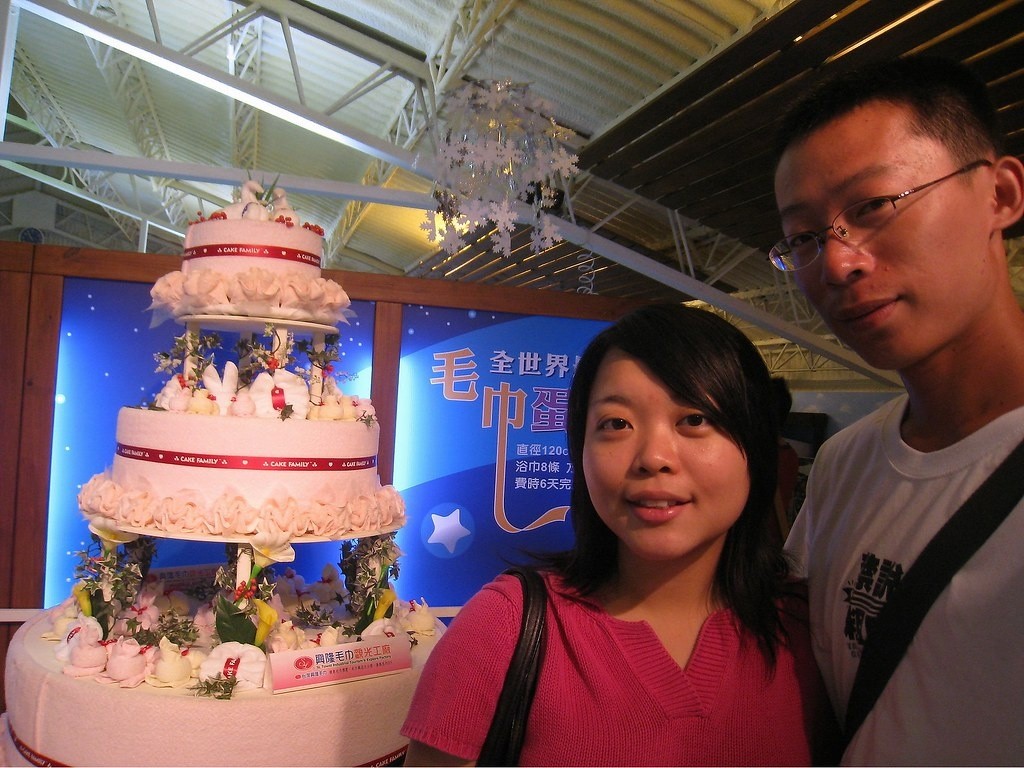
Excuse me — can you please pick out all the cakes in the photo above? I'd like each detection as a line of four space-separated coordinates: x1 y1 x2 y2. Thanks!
5 182 445 768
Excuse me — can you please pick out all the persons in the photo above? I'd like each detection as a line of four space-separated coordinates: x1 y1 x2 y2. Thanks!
767 378 799 545
399 301 813 768
776 52 1024 767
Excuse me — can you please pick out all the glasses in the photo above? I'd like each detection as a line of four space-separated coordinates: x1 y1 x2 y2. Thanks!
765 158 993 273
